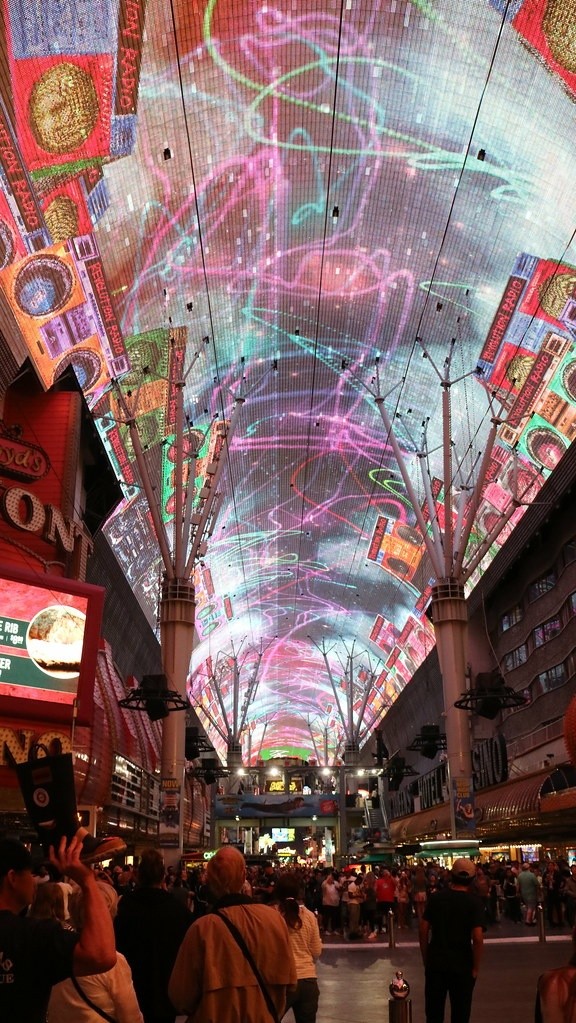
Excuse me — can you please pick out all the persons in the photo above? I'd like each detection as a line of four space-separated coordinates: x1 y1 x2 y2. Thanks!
269 873 323 1023
0 839 576 1023
240 797 314 814
420 857 484 1023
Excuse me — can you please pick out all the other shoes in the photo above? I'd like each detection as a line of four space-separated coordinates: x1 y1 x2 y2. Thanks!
321 922 411 940
512 917 576 929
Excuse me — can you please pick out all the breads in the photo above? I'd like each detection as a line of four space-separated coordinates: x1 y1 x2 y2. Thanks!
28 608 86 674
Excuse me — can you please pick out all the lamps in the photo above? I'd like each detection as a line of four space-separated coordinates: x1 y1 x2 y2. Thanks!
186 758 229 786
379 757 420 791
118 672 192 724
235 815 242 822
311 814 318 821
452 672 530 722
185 726 215 762
371 753 392 761
406 726 448 759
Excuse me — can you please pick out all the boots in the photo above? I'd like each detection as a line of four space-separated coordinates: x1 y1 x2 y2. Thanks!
15 746 126 872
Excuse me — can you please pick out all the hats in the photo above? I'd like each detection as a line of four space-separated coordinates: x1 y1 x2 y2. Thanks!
0 838 44 873
522 863 531 870
454 859 475 878
